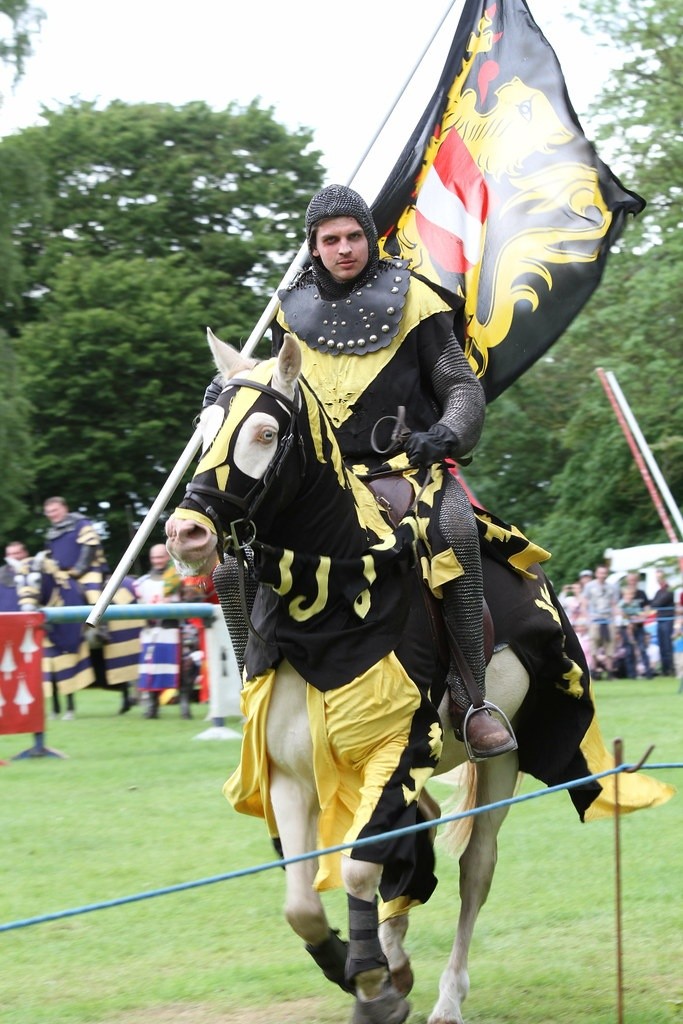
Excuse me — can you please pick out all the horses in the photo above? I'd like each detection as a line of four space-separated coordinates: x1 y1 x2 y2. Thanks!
163 320 531 1016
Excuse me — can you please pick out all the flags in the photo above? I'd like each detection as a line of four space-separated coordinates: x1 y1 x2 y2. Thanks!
368 0 648 401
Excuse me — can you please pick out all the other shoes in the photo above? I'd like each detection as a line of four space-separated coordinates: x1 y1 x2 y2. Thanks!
449 694 515 757
95 624 112 644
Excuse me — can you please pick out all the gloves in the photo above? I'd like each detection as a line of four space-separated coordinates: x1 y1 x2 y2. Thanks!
403 423 461 469
202 379 222 408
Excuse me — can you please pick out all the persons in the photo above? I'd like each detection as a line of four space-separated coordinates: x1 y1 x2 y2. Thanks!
0 541 31 612
212 185 516 758
42 497 111 644
557 563 677 680
132 544 195 720
